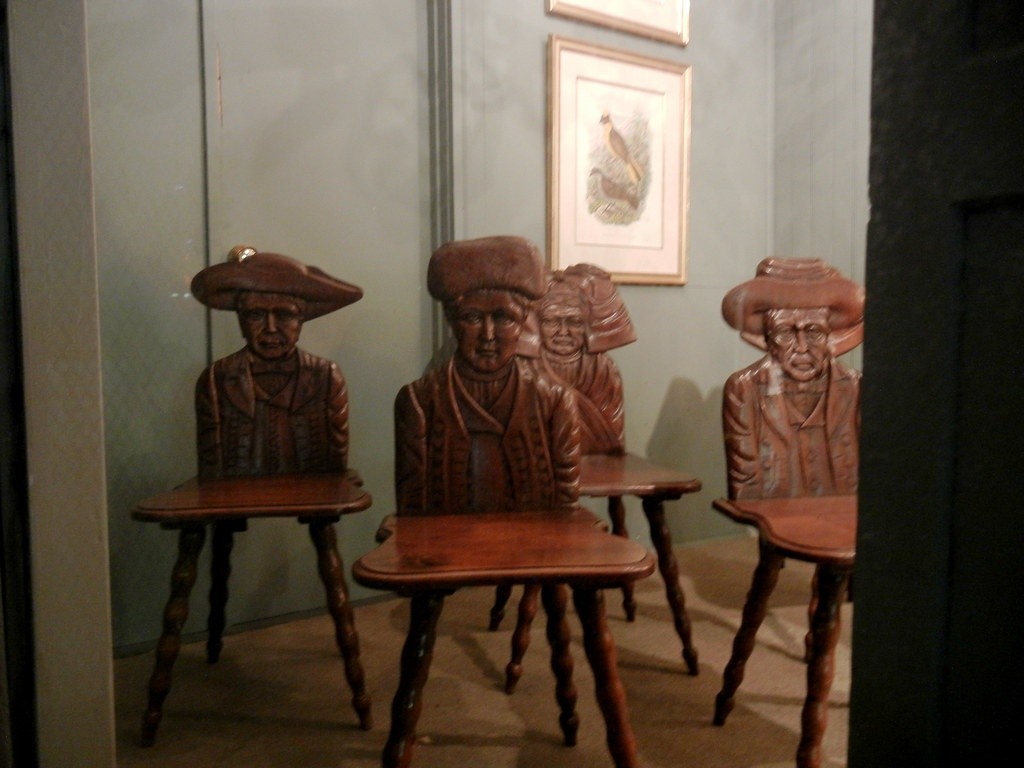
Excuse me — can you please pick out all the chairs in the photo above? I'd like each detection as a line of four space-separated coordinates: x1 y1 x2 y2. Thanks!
713 257 865 768
128 246 373 748
489 262 702 694
350 237 641 768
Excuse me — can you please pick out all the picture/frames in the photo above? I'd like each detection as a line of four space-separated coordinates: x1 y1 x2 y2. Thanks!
544 0 692 45
545 34 692 284
